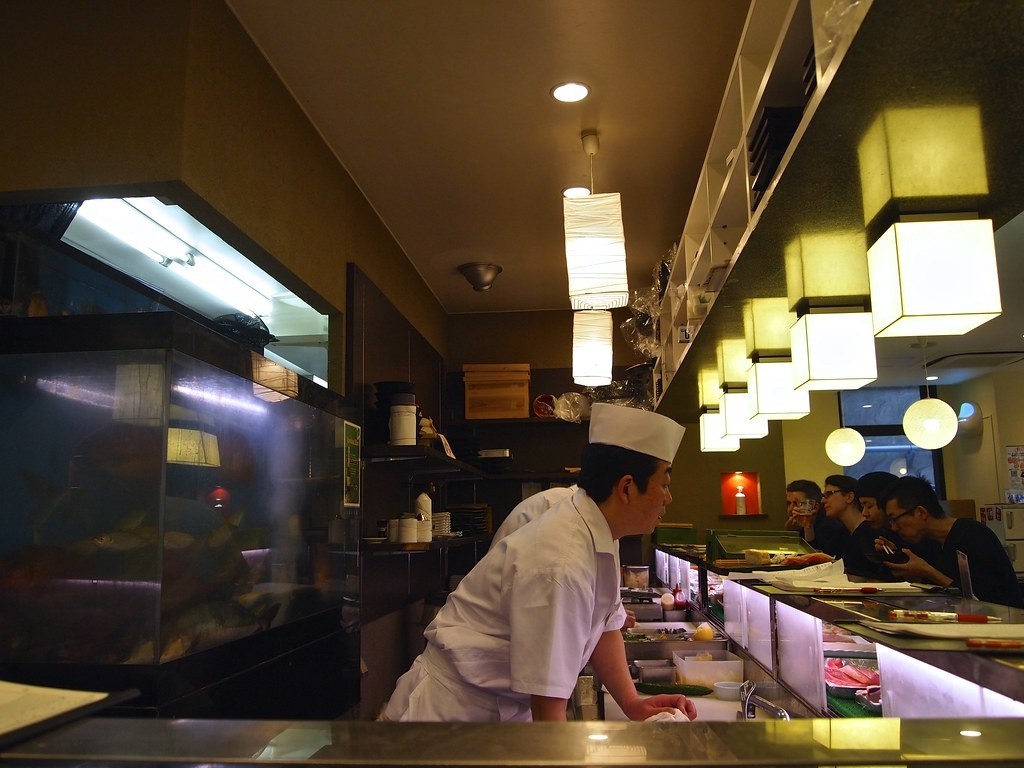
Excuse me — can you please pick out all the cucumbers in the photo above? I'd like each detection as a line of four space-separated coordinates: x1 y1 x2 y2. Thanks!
633 682 714 695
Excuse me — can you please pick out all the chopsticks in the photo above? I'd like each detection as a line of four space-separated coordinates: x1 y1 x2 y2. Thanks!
882 545 894 554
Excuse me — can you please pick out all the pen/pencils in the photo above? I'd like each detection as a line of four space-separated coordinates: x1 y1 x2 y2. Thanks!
813 586 887 595
889 609 1005 625
965 638 1024 650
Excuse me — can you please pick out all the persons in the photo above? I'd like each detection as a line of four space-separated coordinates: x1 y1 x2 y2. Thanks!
784 480 844 552
856 472 946 583
791 475 867 575
874 476 1024 610
482 484 636 628
377 403 697 721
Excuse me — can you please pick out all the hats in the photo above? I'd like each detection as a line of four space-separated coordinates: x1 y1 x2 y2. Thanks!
589 403 686 464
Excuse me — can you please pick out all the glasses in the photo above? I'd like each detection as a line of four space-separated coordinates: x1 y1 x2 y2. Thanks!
885 503 929 525
818 490 841 499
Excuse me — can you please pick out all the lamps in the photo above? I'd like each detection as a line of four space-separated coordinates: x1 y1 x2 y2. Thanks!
572 310 614 387
903 335 959 450
856 103 992 250
742 297 792 359
716 339 754 392
698 370 724 415
866 212 1003 338
783 228 871 317
719 388 768 439
562 130 630 311
788 306 879 390
746 357 811 420
824 391 866 466
699 409 741 453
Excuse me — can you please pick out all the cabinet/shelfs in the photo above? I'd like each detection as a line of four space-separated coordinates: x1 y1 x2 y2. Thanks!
360 445 494 554
650 0 872 412
447 416 590 480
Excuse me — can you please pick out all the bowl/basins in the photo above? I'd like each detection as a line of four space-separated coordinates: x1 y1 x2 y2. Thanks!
715 682 741 701
881 549 914 568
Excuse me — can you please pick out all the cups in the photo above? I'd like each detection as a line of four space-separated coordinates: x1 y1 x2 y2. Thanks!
390 519 399 542
797 498 819 528
400 518 417 543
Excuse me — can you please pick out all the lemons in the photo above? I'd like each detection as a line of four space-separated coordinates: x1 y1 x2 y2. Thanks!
692 627 713 640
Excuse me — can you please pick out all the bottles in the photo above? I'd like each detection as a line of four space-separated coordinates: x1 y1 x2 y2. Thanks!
735 486 746 515
675 590 686 610
416 492 433 542
673 584 680 596
662 593 675 610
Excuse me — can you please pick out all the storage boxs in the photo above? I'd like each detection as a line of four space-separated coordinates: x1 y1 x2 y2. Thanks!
652 522 698 546
672 649 745 690
705 528 818 563
462 363 531 419
1 310 367 700
